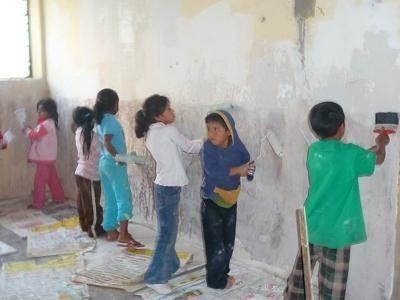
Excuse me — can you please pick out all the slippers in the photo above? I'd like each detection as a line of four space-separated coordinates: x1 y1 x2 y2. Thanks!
115 239 146 248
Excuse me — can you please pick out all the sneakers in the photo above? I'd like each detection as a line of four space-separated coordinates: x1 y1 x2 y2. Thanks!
144 280 172 295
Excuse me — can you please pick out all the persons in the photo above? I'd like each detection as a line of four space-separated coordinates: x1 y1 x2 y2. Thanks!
20 97 68 210
197 108 258 290
134 93 209 296
92 88 146 250
70 106 108 238
282 100 390 300
1 130 8 151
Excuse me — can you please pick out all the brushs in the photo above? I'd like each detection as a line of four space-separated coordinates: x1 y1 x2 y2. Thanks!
247 130 285 166
208 104 234 113
3 129 15 144
373 112 399 136
115 153 146 165
14 108 27 129
